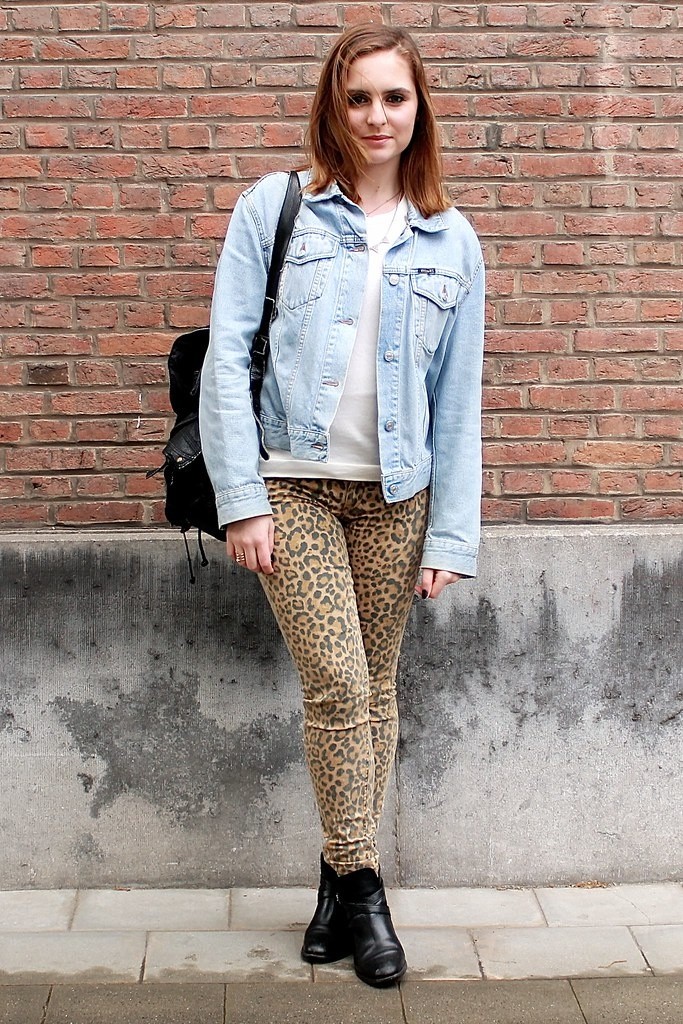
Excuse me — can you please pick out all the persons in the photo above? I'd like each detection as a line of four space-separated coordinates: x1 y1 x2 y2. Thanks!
198 24 486 988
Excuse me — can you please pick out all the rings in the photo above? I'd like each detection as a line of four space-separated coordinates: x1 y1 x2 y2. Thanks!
236 553 245 562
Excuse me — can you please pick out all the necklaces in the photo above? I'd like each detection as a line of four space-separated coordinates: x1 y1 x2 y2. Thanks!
357 191 401 252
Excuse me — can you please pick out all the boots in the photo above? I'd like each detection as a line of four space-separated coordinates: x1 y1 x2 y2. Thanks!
300 851 351 964
335 867 408 984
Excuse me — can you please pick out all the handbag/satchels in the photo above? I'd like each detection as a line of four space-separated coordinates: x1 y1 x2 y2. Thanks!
146 170 303 585
